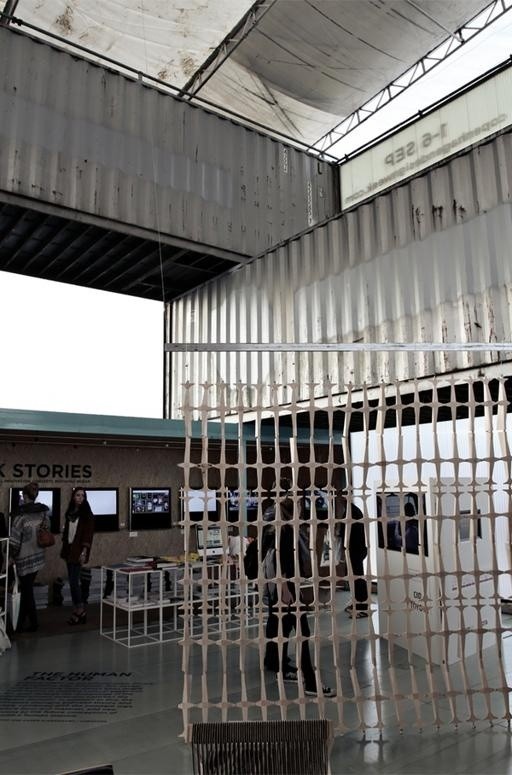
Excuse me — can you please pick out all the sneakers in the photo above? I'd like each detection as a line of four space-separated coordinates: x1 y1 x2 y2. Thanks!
304 685 338 698
348 610 370 618
274 670 300 683
344 605 354 612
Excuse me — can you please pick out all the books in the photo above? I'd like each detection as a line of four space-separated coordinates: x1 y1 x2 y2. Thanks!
31 553 204 610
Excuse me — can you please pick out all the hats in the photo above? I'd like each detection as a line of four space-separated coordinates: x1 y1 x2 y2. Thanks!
23 481 39 498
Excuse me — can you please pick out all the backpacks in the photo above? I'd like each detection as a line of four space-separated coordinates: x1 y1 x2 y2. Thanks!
254 547 296 607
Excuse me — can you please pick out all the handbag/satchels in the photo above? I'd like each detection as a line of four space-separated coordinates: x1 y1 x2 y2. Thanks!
36 524 56 547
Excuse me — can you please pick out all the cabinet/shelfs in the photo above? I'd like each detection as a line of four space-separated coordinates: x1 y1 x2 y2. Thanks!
1 536 9 628
100 552 273 649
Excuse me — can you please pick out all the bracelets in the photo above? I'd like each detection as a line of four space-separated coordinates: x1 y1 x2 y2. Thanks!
82 552 86 556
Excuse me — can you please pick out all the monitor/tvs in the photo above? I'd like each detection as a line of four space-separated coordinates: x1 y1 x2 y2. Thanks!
377 492 428 556
178 487 329 522
129 487 172 530
9 488 61 534
195 525 231 558
460 510 481 540
73 488 120 531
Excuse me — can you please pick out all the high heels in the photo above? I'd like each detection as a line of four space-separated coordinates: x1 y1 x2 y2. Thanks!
68 610 88 625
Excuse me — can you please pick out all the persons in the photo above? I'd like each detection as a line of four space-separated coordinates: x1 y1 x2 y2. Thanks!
260 475 300 674
227 525 251 560
393 502 418 556
1 480 52 634
330 496 372 618
272 497 336 697
57 486 96 629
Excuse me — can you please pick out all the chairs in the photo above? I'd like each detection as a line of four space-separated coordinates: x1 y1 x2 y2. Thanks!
60 763 115 775
190 717 333 774
